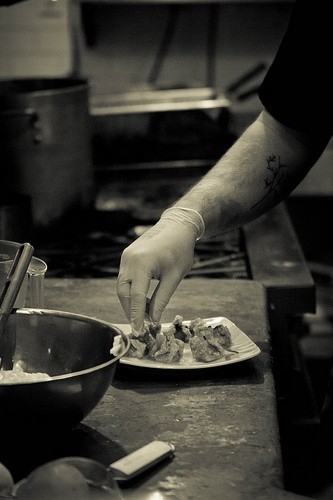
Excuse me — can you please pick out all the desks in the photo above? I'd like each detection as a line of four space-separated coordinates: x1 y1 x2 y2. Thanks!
42 278 285 500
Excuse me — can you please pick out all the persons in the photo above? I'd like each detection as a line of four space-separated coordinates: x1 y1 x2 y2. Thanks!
115 0 333 337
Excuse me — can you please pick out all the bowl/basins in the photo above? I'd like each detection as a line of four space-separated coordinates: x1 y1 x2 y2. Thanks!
0 307 131 437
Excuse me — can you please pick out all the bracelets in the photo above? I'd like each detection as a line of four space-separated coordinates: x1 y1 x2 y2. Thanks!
162 207 205 241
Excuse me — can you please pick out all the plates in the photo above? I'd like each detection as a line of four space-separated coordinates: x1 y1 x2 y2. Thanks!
110 316 262 370
